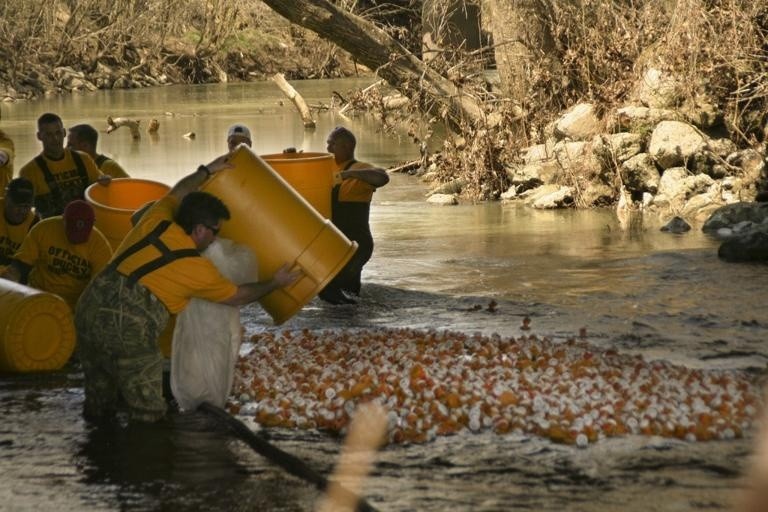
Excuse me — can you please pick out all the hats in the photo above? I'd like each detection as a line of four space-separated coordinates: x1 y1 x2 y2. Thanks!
63 199 95 245
228 124 251 139
7 177 33 205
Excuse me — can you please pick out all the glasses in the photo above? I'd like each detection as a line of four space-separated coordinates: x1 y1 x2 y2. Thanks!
202 222 220 235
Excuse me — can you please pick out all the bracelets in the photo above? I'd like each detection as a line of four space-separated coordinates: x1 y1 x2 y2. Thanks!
197 164 211 179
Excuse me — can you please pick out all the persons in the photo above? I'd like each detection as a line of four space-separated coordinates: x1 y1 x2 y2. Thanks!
73 153 302 425
0 179 42 269
228 125 253 151
20 113 110 221
67 124 131 179
0 199 114 312
283 126 390 307
0 106 14 199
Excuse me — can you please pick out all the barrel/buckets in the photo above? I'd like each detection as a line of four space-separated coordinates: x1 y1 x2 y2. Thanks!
0 277 76 373
84 178 173 253
200 143 358 324
256 151 338 226
